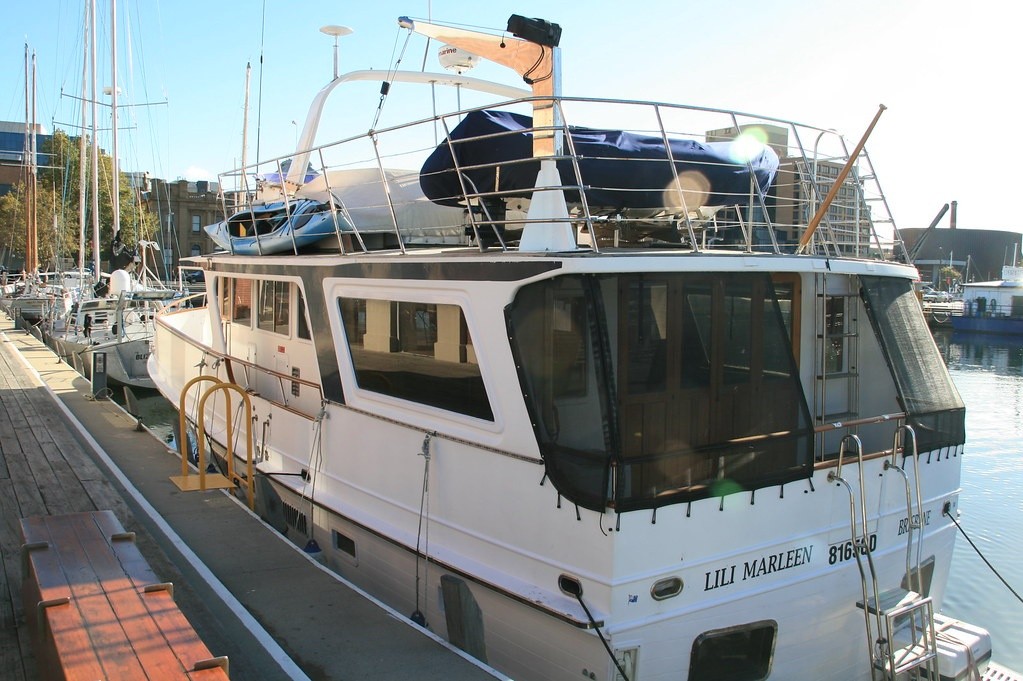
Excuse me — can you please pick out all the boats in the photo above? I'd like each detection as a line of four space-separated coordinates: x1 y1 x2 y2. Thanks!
1 17 1023 680
